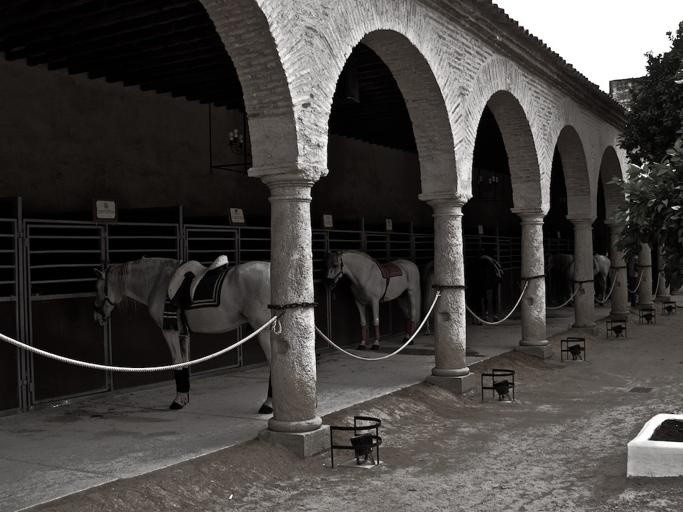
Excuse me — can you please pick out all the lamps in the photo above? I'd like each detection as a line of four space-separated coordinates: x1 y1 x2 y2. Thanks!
488 176 498 185
228 129 244 155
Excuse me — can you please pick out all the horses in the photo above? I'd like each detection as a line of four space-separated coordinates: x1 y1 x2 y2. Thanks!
91 255 273 415
465 248 500 324
545 252 612 307
422 260 434 336
326 248 422 351
